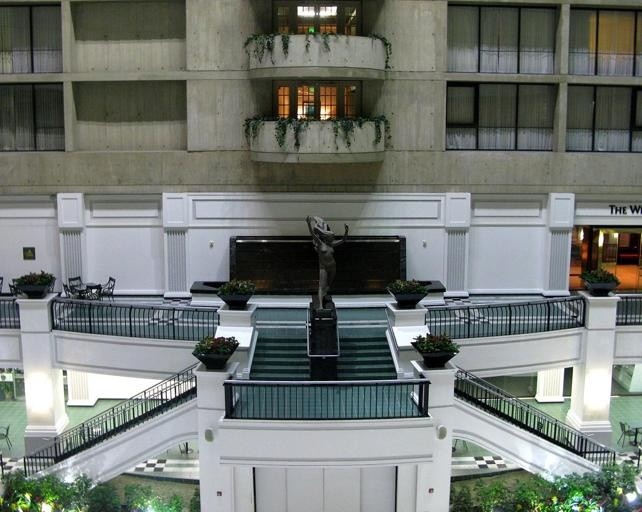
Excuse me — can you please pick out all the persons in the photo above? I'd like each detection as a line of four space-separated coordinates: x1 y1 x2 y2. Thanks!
304 215 349 309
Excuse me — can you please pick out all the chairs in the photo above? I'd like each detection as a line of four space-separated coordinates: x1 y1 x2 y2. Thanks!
0 424 13 450
62 275 116 302
616 420 637 447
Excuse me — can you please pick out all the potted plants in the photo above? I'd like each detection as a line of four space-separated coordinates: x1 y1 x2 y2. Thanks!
577 269 620 296
12 269 57 298
192 336 240 369
216 281 256 310
409 331 462 367
386 279 429 309
